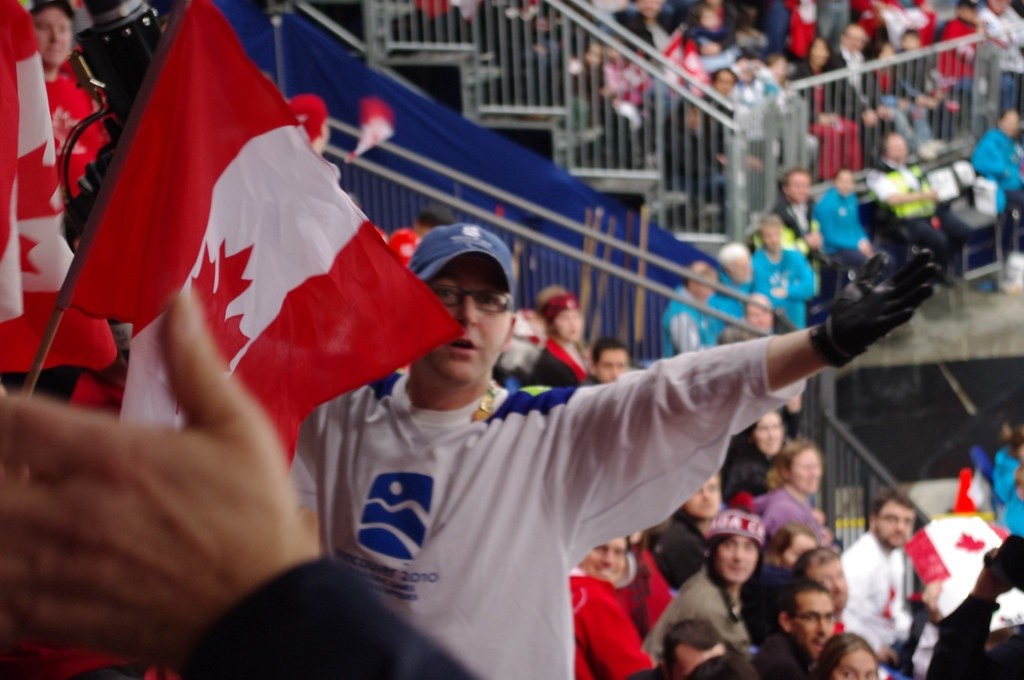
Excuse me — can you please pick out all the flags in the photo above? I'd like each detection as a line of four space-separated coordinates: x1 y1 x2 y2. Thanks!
66 0 466 472
2 1 115 372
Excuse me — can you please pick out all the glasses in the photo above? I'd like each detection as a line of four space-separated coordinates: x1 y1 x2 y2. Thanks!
793 612 836 625
427 283 515 312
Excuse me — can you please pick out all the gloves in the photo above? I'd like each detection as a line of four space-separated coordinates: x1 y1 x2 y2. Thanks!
808 248 945 369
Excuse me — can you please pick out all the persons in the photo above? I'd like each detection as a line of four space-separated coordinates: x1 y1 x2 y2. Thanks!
570 392 1024 680
389 0 1023 265
5 289 480 680
503 112 1021 391
27 1 112 196
288 221 941 680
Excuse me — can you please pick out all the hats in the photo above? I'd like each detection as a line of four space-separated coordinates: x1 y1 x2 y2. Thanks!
702 508 766 550
409 222 514 294
